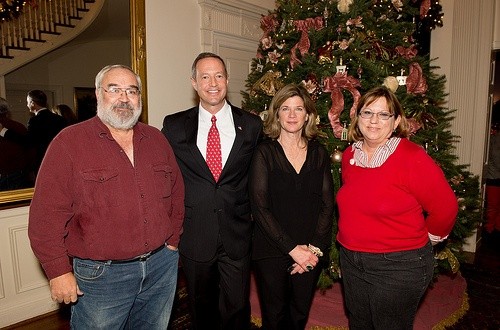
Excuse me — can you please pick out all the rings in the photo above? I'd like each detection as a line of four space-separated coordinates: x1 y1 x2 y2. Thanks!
307 265 313 270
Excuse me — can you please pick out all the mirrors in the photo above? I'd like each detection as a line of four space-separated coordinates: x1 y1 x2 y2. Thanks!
0 0 148 213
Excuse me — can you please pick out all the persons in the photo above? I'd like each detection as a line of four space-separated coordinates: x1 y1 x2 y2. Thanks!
28 65 185 330
162 53 266 330
336 88 458 330
0 90 81 193
251 85 334 330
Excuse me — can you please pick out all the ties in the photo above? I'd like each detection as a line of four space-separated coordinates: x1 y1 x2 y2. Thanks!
206 115 223 184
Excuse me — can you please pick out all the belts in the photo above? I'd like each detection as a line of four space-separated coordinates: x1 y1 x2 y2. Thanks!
95 243 165 264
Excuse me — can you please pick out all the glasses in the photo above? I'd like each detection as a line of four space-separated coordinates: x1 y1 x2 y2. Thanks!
101 87 141 99
359 108 395 121
